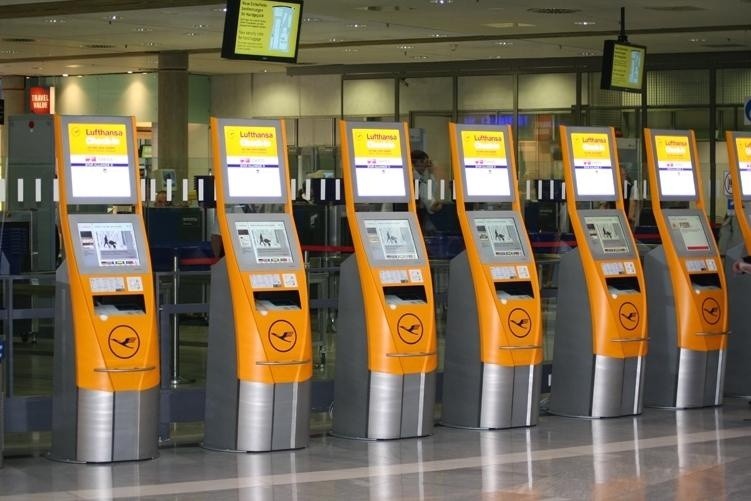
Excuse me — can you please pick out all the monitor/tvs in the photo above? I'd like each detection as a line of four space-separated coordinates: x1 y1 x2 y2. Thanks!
725 130 751 201
448 122 521 203
209 117 292 204
52 114 143 206
600 39 647 93
339 119 416 203
643 127 704 202
220 0 305 64
559 124 623 202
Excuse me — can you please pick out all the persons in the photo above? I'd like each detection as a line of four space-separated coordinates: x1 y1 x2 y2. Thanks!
210 204 222 259
411 150 447 199
155 189 170 208
620 165 637 201
